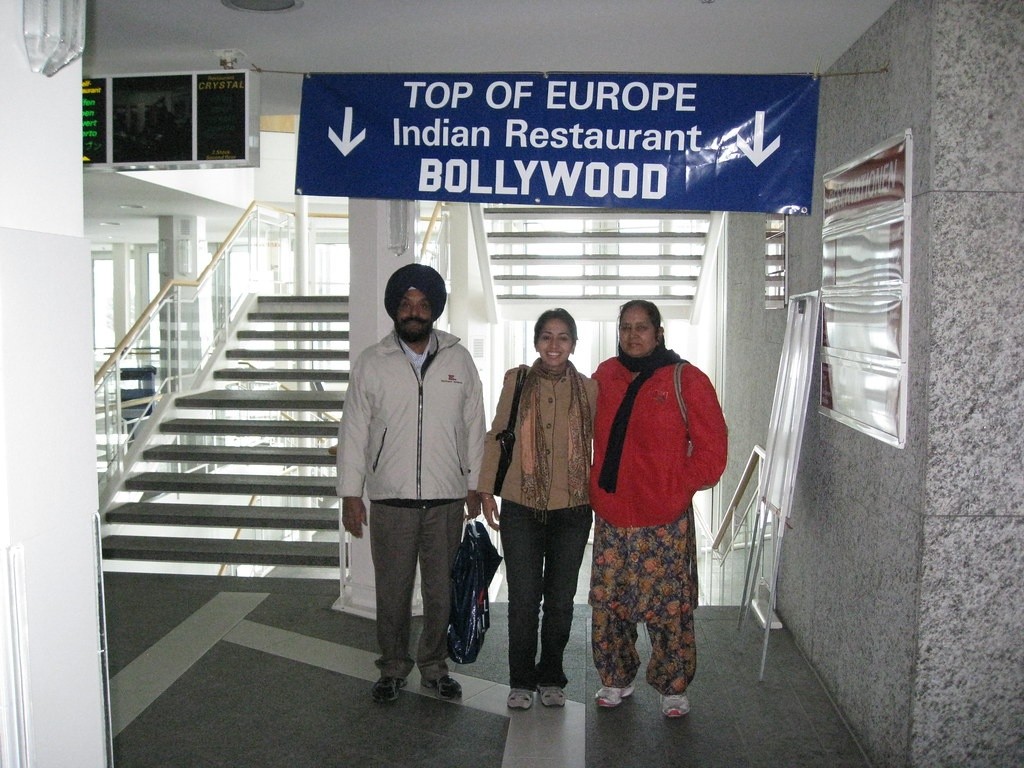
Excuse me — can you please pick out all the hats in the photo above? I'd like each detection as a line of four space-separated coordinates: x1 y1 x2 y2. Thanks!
384 263 447 319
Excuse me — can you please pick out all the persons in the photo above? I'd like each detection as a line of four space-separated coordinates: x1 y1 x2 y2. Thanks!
336 263 486 704
588 300 728 717
475 308 599 710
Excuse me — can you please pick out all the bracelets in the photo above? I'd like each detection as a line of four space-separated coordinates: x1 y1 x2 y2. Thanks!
478 493 494 499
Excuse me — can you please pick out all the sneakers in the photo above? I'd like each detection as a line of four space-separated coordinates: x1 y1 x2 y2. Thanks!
536 684 566 708
506 687 534 709
594 678 636 707
660 690 691 718
420 673 462 700
372 676 409 701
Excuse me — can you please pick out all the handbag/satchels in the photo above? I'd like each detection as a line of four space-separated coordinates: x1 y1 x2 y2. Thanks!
494 429 516 498
445 521 504 664
